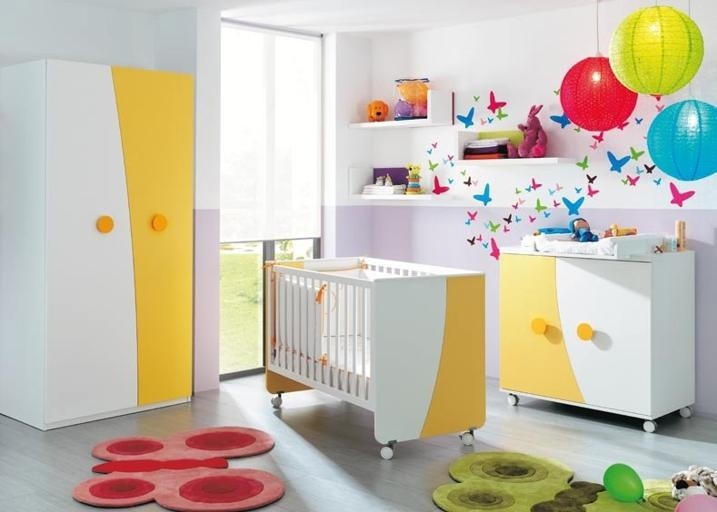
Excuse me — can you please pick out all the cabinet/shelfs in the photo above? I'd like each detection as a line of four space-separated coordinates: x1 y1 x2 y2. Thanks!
498 249 696 433
347 89 575 200
0 59 194 432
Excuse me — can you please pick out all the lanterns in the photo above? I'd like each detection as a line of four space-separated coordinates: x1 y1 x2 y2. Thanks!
558 57 638 132
608 5 704 96
646 98 717 182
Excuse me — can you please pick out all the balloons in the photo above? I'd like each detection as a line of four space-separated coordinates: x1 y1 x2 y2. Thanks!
603 463 646 503
674 494 717 511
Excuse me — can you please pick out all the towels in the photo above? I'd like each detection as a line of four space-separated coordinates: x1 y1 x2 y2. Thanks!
536 233 660 255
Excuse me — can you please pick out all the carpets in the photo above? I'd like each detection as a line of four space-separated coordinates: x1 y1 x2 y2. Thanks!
72 426 284 512
433 451 673 512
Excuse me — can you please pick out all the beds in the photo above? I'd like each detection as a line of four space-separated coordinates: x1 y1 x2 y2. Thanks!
264 258 486 459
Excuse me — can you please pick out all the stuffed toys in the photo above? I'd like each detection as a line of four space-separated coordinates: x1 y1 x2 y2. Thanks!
569 216 599 242
515 104 548 158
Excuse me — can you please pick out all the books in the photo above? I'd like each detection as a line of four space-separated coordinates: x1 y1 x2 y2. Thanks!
363 183 405 190
463 129 526 159
361 189 404 194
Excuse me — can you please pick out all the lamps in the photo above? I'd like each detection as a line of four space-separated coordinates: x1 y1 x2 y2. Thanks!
561 0 717 182
